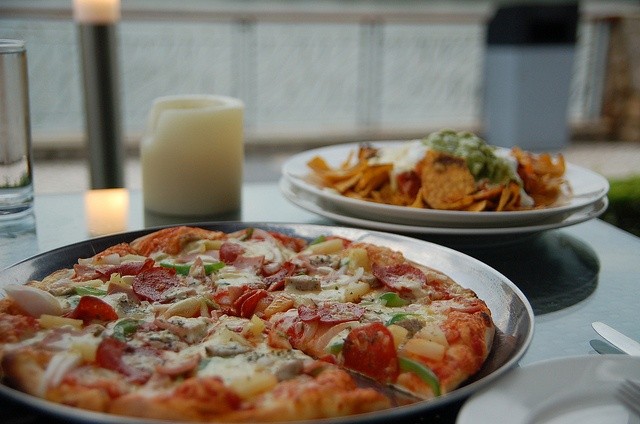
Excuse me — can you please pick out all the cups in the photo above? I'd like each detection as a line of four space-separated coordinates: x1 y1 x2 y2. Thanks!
0 39 34 215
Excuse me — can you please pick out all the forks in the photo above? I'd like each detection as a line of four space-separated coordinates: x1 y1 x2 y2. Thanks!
613 378 640 415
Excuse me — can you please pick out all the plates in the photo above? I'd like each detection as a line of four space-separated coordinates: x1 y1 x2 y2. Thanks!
456 355 640 424
281 187 609 248
281 139 611 226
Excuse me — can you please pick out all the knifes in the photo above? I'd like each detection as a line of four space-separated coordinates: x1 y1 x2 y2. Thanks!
591 321 640 356
